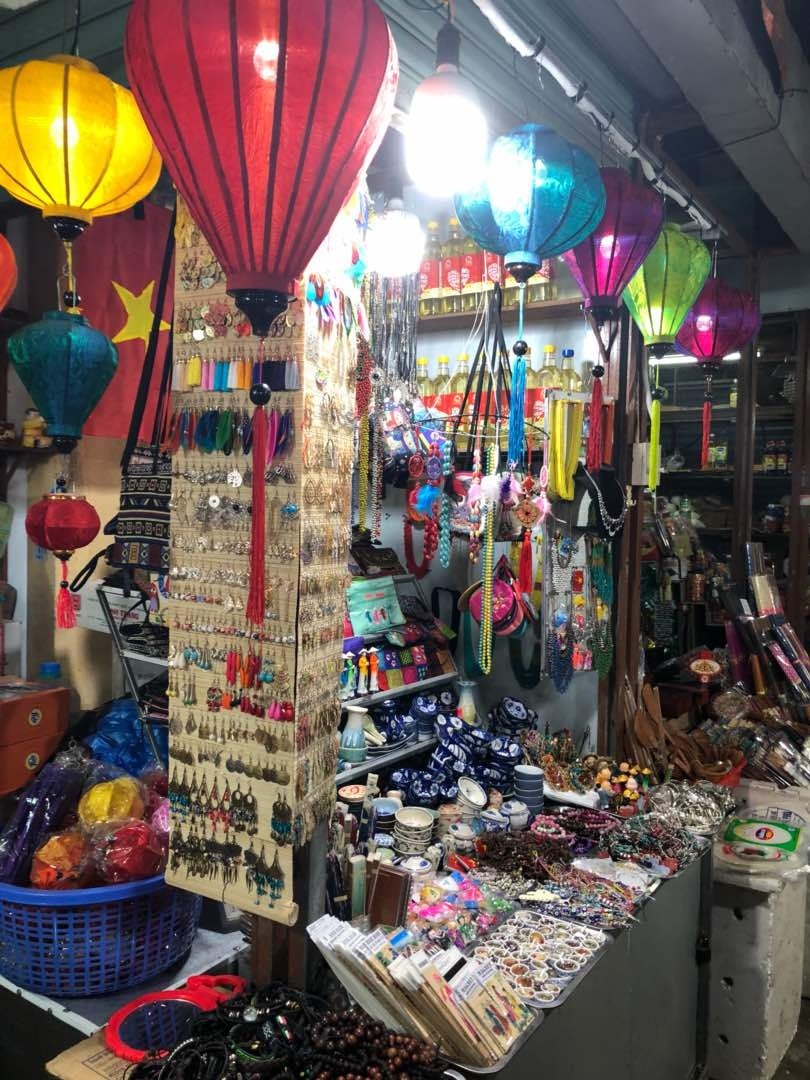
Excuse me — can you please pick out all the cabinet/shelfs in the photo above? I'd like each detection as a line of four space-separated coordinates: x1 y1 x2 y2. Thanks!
370 294 601 465
648 374 752 614
753 397 802 579
335 568 467 786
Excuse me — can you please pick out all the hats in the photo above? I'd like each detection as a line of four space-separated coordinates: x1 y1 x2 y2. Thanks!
468 579 524 636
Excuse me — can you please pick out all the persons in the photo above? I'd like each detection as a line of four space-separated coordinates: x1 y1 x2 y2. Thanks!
340 645 380 699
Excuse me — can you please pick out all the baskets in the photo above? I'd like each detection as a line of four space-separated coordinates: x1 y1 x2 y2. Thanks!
0 875 203 997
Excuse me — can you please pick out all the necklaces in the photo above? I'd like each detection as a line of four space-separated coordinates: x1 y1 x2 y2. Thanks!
479 445 496 675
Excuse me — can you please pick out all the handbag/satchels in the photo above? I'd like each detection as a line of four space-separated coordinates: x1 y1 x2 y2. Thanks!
110 445 172 575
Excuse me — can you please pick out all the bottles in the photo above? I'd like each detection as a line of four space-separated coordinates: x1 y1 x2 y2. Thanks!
707 433 727 470
32 661 81 731
762 503 791 533
419 214 556 313
762 440 789 470
376 700 407 742
679 498 692 522
338 706 370 764
729 378 738 408
414 345 582 448
456 679 480 724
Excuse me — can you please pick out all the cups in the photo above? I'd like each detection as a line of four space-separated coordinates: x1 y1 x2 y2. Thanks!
688 570 705 601
400 856 433 904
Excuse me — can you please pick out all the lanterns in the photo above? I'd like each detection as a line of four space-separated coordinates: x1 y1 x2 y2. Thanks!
0 53 162 240
122 0 399 624
453 120 607 464
0 743 207 998
555 166 667 475
8 310 119 455
25 475 101 629
672 276 758 464
618 209 712 492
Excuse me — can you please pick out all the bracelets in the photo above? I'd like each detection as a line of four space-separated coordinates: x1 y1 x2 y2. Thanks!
127 977 449 1080
466 766 740 936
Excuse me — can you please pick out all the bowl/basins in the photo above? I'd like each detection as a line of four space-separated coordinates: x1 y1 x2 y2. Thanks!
370 690 545 862
